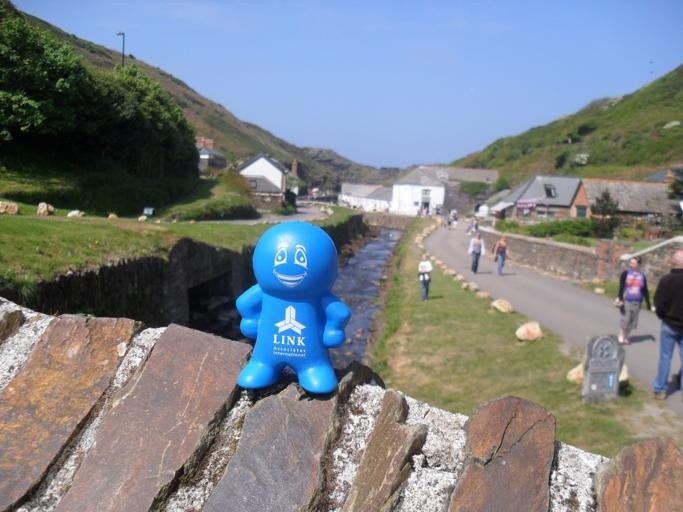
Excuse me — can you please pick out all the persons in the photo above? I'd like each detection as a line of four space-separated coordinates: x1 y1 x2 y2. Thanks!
418 254 433 304
467 218 485 275
494 235 513 277
615 249 683 399
421 206 459 231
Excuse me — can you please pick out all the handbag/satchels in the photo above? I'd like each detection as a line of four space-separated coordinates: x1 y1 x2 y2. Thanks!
613 296 624 307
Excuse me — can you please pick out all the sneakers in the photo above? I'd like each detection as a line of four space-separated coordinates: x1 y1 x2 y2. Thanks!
652 387 669 399
617 334 630 345
